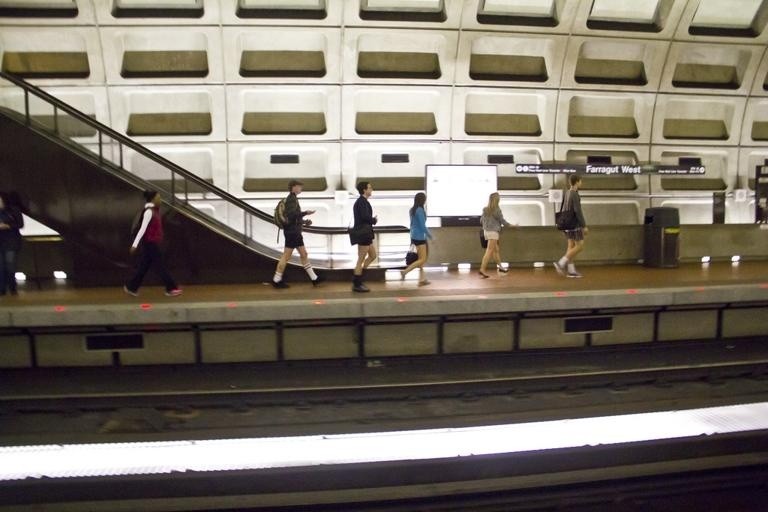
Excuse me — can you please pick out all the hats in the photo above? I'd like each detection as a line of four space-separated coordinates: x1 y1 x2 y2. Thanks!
287 178 305 188
143 191 158 203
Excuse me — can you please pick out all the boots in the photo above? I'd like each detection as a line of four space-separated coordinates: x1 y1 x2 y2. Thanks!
351 267 372 294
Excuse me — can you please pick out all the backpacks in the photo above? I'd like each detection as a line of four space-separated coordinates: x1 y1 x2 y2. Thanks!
272 193 308 244
127 205 153 255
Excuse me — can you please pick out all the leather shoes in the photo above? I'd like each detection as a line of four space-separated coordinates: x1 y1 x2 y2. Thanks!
496 263 509 274
477 270 491 279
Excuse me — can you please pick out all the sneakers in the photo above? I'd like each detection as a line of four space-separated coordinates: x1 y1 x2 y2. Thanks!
565 270 585 279
309 270 329 287
552 260 563 275
416 279 432 288
399 269 408 282
0 287 7 296
163 287 184 297
269 278 291 290
8 286 18 297
121 282 141 298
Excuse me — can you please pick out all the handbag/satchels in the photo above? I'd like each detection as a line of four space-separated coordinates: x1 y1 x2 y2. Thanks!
554 209 579 231
404 243 418 266
479 227 488 248
347 210 377 247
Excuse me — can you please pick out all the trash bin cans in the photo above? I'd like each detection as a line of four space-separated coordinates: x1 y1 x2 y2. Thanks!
642 207 680 268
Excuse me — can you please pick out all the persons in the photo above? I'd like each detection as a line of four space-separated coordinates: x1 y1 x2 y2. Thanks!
554 174 590 278
400 192 434 287
353 181 378 292
0 191 24 296
478 193 517 278
122 190 185 298
268 183 327 289
